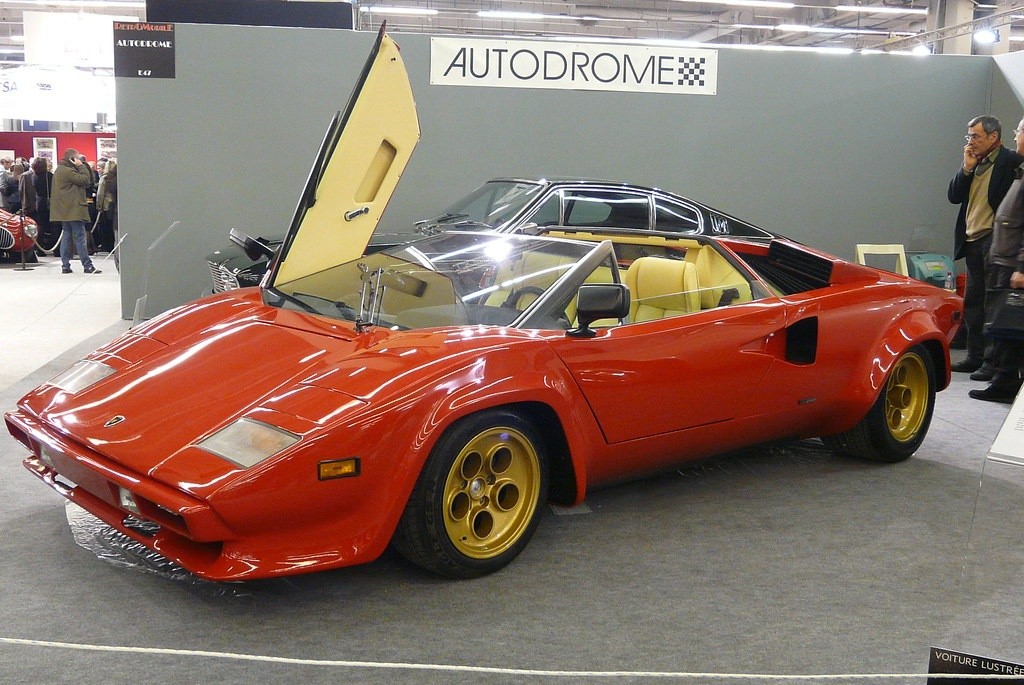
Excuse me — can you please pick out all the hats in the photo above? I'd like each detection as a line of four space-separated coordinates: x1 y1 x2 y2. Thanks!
78 154 87 163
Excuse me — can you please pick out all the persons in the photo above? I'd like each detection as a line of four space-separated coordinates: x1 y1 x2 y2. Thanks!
947 115 1024 382
0 155 117 257
50 148 102 274
968 118 1024 406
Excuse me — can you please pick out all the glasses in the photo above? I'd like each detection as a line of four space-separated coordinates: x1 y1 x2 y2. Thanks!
1012 129 1024 138
965 132 991 141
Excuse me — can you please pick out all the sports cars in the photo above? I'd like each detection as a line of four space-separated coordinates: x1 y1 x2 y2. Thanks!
3 28 965 582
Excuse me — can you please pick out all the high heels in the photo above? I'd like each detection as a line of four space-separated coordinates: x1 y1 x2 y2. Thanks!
88 250 98 255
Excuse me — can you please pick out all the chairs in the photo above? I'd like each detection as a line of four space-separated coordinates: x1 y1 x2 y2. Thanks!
623 257 701 324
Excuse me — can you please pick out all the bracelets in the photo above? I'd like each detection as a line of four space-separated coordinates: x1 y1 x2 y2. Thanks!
963 166 973 172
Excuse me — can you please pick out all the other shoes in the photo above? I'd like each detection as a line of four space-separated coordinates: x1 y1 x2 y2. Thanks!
62 265 73 273
970 365 999 381
54 251 60 257
951 353 981 373
970 379 1021 404
37 252 46 257
84 264 102 273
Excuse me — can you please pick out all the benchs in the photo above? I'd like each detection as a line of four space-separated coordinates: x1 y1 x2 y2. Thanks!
484 250 629 326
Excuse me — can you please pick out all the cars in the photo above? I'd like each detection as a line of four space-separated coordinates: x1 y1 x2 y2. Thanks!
0 207 38 263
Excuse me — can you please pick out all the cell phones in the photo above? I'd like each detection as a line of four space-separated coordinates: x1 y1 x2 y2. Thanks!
71 157 74 161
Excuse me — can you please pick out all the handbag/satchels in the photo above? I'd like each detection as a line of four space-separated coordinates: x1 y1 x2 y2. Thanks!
46 198 51 209
983 278 1024 341
